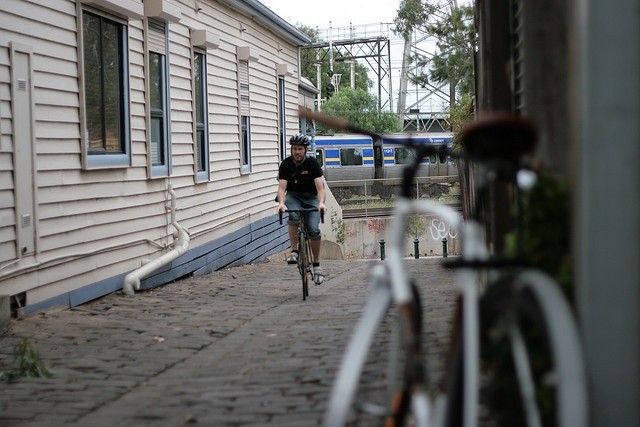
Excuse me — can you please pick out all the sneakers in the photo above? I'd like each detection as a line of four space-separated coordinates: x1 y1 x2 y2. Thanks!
314 266 325 285
287 252 299 262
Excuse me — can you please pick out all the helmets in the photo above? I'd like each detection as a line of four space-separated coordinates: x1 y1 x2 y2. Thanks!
289 133 309 145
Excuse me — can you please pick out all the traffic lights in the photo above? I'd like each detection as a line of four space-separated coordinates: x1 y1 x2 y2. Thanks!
327 70 333 76
326 84 335 100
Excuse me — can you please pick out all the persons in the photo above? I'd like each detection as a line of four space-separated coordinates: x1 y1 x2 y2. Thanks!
276 135 326 284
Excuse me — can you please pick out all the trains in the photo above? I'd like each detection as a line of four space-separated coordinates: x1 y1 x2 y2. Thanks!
306 132 459 201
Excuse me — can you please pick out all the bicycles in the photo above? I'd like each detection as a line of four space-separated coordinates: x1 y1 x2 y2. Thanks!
299 107 589 426
279 207 324 299
